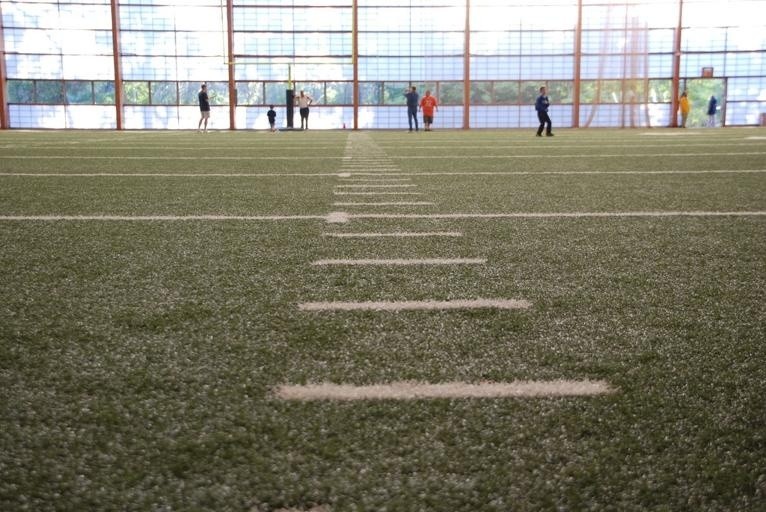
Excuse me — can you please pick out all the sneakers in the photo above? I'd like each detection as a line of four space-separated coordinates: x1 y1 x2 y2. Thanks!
271 129 275 132
537 132 554 137
409 128 432 132
301 127 309 129
198 129 208 133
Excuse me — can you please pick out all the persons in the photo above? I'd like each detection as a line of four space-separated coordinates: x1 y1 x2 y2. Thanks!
196 84 215 134
402 86 420 132
266 104 276 133
292 90 313 130
416 89 439 131
678 88 691 128
705 96 718 128
534 87 555 136
